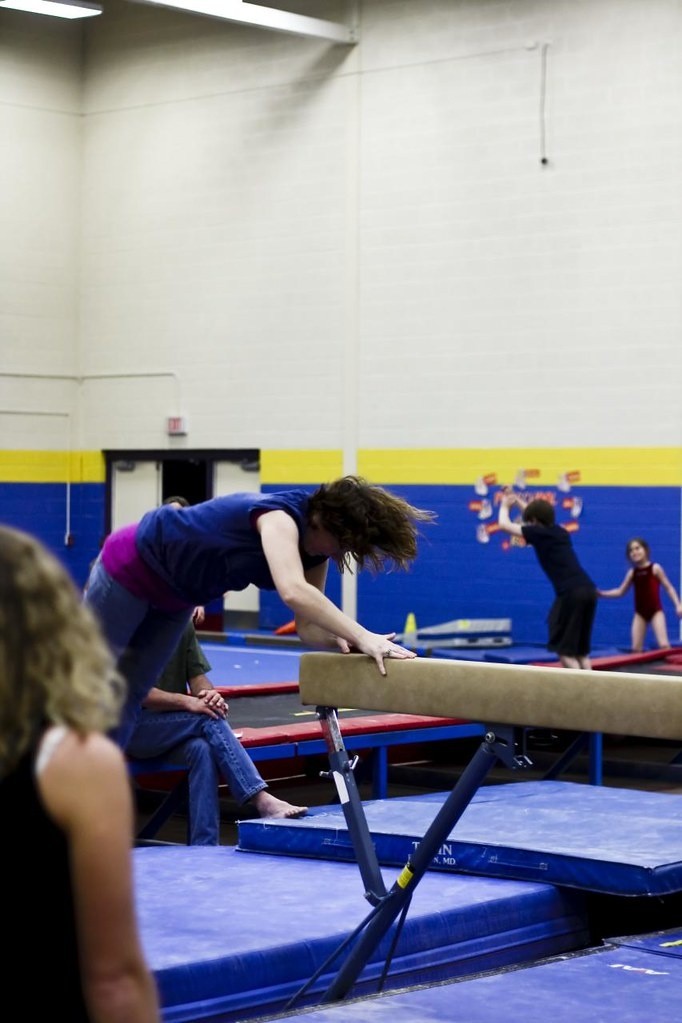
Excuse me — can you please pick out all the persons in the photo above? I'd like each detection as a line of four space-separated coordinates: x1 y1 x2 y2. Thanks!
84 475 435 749
0 525 159 1023
130 621 308 846
498 488 597 670
168 497 205 624
596 539 682 653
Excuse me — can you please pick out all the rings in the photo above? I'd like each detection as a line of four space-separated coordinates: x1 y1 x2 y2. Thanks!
386 649 391 657
